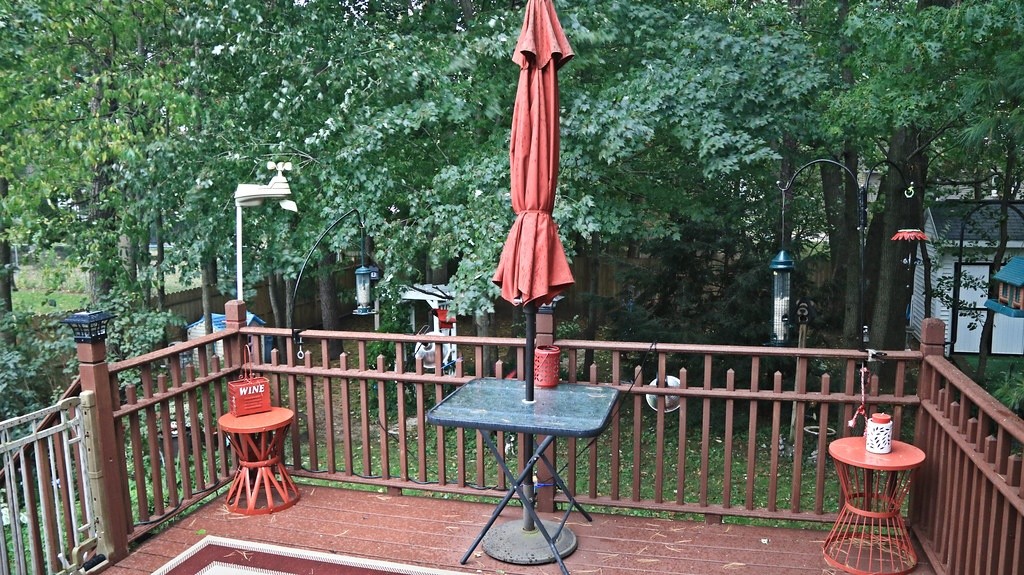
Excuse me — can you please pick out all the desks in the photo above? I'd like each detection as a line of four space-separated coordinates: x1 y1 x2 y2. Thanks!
427 378 619 575
218 406 301 516
821 437 926 575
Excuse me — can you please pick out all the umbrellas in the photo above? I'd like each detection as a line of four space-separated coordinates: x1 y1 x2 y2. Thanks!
492 0 575 531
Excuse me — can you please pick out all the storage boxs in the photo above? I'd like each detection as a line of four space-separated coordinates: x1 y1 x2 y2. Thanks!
228 375 271 417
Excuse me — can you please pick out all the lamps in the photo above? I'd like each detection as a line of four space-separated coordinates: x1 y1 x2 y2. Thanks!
645 375 681 413
765 250 794 345
352 266 378 315
59 303 118 344
